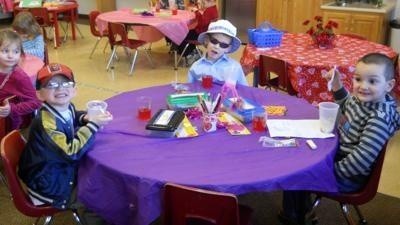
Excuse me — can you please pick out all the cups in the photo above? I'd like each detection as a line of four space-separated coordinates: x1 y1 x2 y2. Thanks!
202 72 214 89
155 6 181 16
252 111 266 134
137 96 153 119
87 100 108 132
204 114 217 133
318 101 340 134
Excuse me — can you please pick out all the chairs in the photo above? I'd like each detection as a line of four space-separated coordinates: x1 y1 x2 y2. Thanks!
259 55 287 91
13 0 219 76
305 125 400 225
161 182 240 225
0 129 82 224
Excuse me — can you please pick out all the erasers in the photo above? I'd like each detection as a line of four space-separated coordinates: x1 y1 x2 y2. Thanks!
306 139 318 150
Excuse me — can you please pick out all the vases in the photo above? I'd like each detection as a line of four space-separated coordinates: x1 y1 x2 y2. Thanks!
319 44 328 49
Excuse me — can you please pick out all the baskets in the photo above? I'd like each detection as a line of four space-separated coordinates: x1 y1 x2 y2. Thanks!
248 25 284 48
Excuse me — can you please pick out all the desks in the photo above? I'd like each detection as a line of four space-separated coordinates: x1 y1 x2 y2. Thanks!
75 78 341 225
240 33 400 106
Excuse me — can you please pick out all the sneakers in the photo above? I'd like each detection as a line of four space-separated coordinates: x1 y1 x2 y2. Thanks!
278 206 319 222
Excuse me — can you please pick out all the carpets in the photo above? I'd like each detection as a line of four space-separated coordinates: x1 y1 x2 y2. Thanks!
0 167 400 225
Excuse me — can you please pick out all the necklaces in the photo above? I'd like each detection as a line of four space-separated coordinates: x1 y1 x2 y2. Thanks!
0 70 14 90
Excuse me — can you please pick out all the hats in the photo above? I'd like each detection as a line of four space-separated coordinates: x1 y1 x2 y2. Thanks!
198 18 242 55
36 62 74 85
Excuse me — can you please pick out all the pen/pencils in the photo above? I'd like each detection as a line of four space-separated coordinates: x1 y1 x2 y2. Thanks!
197 93 224 113
3 95 17 101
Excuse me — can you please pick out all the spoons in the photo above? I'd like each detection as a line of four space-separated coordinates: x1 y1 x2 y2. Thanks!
328 65 337 92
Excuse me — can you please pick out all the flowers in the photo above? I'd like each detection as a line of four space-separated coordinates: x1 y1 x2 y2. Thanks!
302 16 339 44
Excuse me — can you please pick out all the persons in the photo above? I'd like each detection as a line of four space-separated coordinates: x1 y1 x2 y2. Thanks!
177 1 218 56
188 19 249 87
13 12 45 62
18 64 112 211
278 53 400 224
0 30 41 130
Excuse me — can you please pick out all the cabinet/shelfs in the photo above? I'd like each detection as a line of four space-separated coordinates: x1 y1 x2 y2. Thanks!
321 8 396 48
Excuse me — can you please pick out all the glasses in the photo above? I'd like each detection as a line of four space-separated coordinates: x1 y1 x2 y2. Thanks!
43 80 76 90
208 34 233 49
